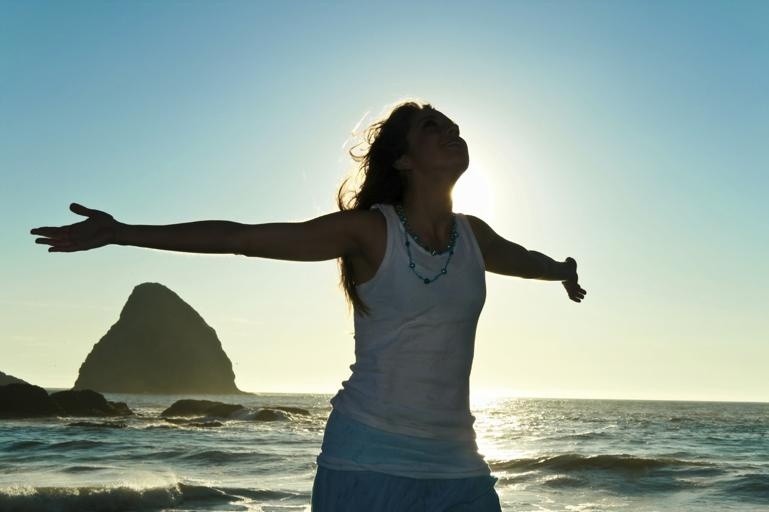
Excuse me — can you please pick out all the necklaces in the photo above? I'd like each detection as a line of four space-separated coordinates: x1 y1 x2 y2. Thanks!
396 202 460 283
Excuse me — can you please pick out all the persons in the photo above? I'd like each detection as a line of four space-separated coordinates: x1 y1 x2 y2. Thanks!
31 101 586 512
